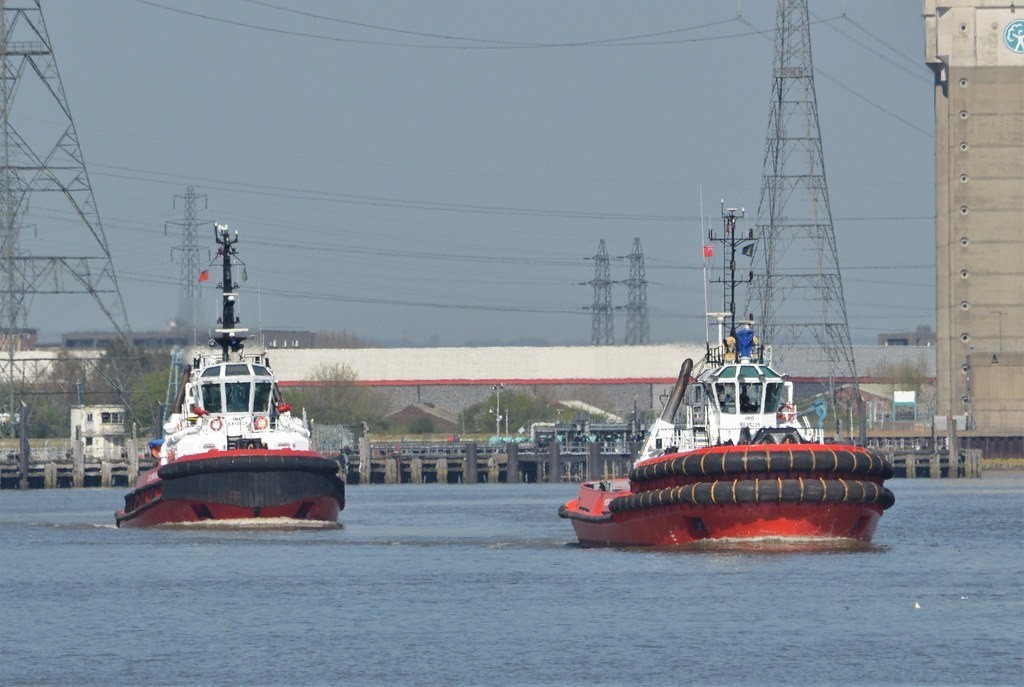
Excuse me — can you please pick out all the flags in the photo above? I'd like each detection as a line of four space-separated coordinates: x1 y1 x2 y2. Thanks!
199 268 209 281
242 268 247 281
741 244 755 257
726 220 730 234
703 245 713 257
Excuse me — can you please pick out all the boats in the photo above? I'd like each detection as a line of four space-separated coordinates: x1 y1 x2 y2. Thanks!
558 196 895 556
110 221 347 531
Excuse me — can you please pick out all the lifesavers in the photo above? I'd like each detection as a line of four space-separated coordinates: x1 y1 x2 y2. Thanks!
777 404 793 421
255 416 268 429
210 418 223 431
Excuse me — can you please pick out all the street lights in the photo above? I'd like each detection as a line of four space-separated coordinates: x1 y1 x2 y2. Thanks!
488 382 504 437
